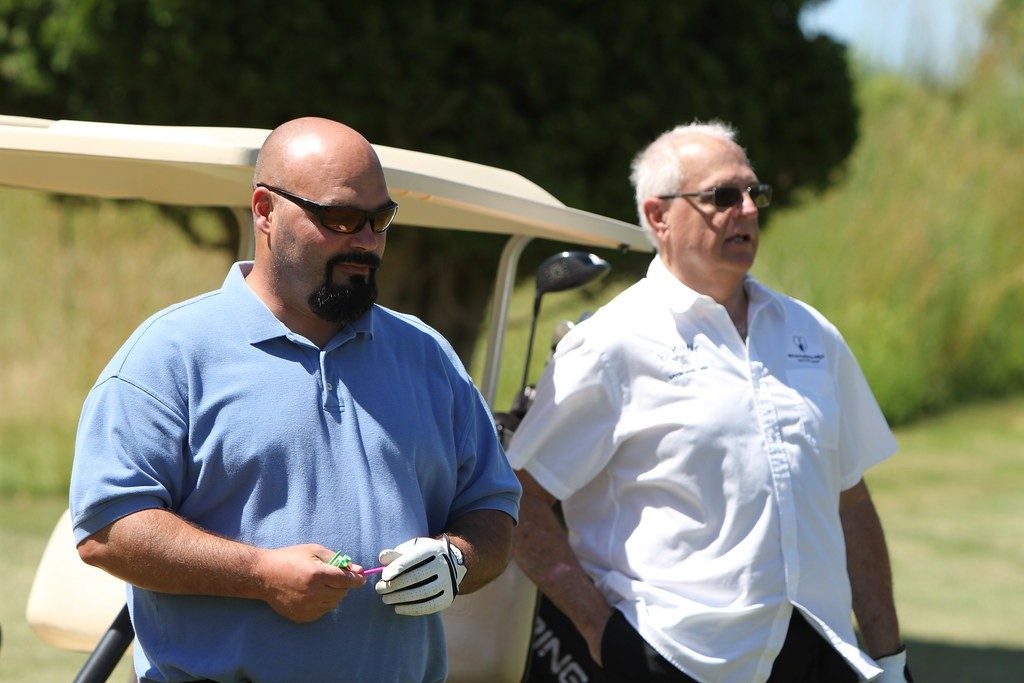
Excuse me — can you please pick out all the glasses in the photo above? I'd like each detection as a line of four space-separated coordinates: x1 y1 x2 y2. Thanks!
257 183 399 234
658 185 773 207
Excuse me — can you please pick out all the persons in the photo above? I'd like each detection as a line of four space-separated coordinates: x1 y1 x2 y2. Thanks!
505 121 922 683
68 116 520 683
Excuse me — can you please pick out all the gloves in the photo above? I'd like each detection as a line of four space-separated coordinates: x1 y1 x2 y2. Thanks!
872 643 915 683
375 532 468 616
